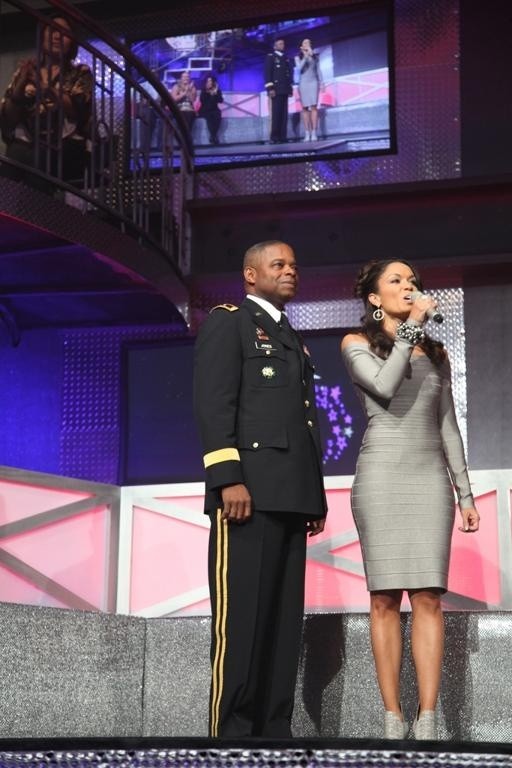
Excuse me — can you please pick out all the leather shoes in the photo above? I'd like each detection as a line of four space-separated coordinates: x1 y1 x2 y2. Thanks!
413 703 437 740
310 130 317 141
384 702 409 739
304 131 311 142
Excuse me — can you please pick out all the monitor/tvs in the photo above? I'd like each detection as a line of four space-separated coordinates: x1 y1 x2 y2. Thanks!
126 0 399 179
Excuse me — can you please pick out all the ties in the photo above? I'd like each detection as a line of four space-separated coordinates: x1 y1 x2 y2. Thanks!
280 311 292 332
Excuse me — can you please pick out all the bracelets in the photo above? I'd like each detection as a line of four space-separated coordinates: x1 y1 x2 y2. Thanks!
395 321 427 346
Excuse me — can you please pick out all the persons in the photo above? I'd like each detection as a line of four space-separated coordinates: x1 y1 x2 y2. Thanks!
262 36 295 144
1 9 102 200
189 238 330 738
197 74 224 145
135 64 171 154
338 257 482 741
293 35 327 143
168 70 197 152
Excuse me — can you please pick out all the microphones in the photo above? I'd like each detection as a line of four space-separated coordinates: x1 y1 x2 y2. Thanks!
410 289 444 329
307 46 314 59
